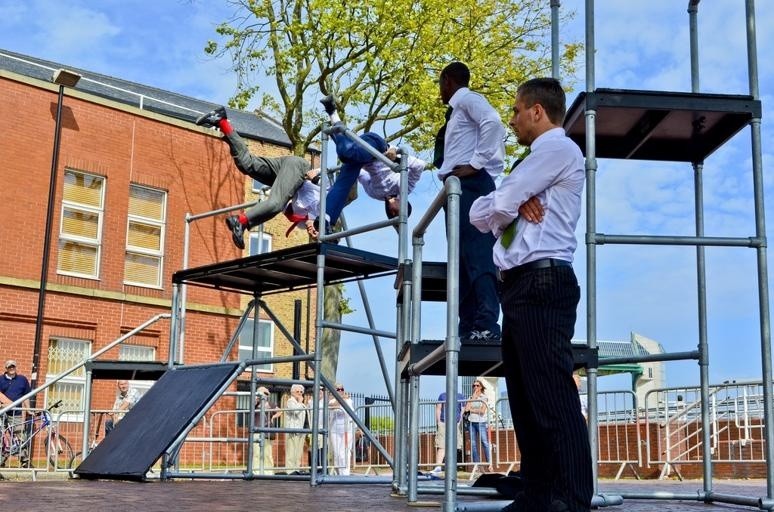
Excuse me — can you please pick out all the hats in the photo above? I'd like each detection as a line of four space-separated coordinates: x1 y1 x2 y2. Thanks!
4 360 16 369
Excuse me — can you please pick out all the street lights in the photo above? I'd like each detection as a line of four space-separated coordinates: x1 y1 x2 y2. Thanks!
26 69 82 465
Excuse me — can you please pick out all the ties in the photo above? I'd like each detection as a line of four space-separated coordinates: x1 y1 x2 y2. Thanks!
285 204 308 237
501 147 532 250
433 106 454 170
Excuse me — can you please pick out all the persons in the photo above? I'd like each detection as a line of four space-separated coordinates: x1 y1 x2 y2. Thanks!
468 78 593 512
434 62 506 341
252 383 357 475
195 105 329 249
432 376 496 473
571 374 589 425
0 360 34 468
108 379 141 422
314 94 425 244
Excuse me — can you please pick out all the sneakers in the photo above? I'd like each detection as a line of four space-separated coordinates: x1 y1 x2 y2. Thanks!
196 106 226 127
226 215 244 249
534 500 591 512
501 500 543 512
314 217 338 244
460 328 500 343
320 94 336 108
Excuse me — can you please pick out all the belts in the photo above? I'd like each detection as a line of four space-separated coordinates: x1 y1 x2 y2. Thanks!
499 258 571 280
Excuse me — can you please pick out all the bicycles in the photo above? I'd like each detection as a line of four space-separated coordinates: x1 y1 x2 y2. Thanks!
0 400 109 480
